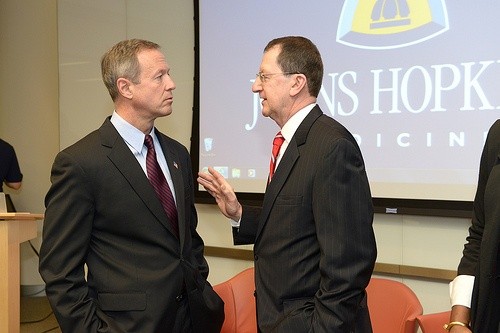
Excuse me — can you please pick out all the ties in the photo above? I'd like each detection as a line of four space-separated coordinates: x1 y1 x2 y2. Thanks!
267 132 286 189
144 134 180 242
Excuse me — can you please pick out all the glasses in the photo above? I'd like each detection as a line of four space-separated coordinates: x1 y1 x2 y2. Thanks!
255 72 300 83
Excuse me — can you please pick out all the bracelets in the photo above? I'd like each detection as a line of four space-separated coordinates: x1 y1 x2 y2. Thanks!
444 322 471 332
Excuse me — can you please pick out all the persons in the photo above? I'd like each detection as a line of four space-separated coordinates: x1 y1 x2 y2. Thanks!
0 139 23 193
37 39 225 333
443 118 500 333
197 36 377 333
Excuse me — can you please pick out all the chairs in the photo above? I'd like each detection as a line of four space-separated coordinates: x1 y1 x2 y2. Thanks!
213 268 423 333
